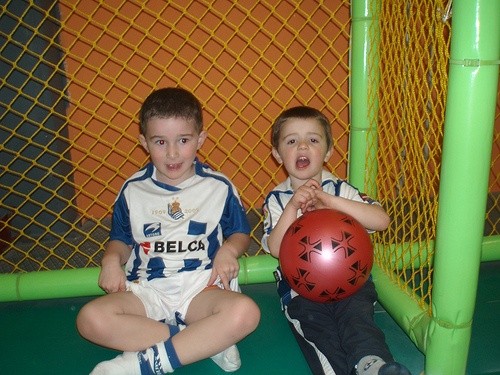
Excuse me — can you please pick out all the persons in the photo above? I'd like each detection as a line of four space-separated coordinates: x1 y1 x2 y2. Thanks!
77 88 259 375
260 105 414 375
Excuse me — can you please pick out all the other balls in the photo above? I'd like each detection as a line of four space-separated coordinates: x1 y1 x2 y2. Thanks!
278 208 374 302
